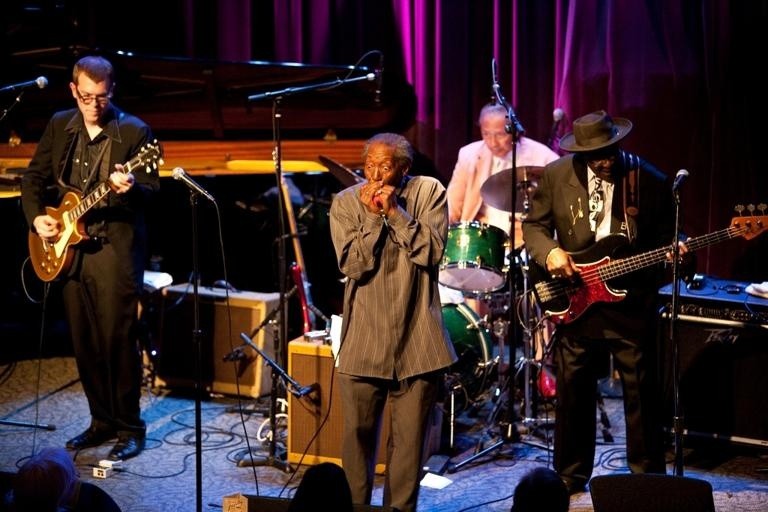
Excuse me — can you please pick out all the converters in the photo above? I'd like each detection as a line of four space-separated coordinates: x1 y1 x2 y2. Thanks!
423 455 451 475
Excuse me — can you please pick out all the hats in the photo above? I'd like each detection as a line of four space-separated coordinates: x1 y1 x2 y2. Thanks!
559 111 632 152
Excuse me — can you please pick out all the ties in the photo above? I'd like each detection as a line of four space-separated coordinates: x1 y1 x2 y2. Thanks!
588 176 604 236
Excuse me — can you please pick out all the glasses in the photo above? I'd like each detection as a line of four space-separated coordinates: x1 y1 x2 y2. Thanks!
76 89 110 104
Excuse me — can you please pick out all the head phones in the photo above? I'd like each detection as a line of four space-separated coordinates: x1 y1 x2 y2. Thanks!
686 270 719 296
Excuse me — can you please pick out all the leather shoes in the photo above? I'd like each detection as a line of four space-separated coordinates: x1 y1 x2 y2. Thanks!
66 419 145 460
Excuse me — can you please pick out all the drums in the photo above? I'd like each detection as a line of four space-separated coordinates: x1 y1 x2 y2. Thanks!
442 302 497 396
438 221 509 293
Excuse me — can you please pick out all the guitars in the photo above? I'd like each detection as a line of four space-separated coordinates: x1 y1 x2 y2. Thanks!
529 205 767 326
28 138 164 281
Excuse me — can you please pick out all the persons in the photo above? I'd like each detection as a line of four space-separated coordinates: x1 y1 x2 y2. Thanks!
328 132 461 512
286 463 354 512
3 452 81 512
443 101 563 398
22 56 163 461
522 109 698 497
510 466 570 512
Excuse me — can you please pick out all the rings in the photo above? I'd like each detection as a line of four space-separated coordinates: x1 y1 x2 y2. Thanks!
364 191 370 195
377 189 383 195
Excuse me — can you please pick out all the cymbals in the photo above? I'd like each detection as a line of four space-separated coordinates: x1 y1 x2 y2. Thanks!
481 166 546 212
319 152 365 186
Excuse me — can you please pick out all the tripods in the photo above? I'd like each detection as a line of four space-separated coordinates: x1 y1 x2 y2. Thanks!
446 145 555 473
237 367 298 474
490 242 556 442
226 108 289 423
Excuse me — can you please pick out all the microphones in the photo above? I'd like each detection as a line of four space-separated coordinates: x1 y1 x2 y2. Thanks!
490 82 500 106
371 55 384 107
172 167 216 203
223 349 258 362
0 76 48 95
373 194 386 218
672 169 689 192
547 108 562 144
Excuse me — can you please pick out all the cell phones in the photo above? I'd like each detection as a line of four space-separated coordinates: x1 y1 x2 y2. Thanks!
727 285 740 293
